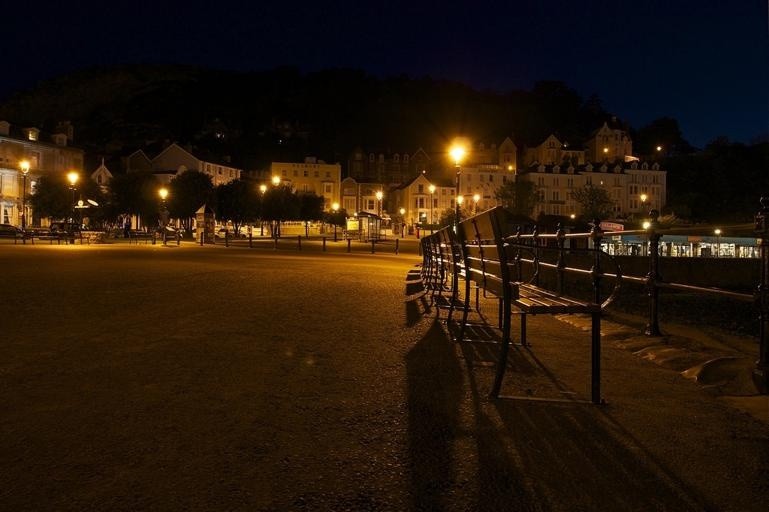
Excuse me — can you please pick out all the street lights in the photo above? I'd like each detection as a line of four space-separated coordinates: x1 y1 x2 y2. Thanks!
157 188 169 246
257 175 282 238
66 171 78 244
18 159 30 231
375 191 384 217
429 135 480 235
399 208 406 238
331 202 340 242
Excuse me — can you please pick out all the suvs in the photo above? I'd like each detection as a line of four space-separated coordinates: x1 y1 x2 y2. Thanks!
49 222 90 232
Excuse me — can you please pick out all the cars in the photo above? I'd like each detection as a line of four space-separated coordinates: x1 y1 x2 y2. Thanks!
175 228 197 240
215 229 250 240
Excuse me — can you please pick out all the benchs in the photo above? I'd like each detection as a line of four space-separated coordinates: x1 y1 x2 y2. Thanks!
417 204 625 406
